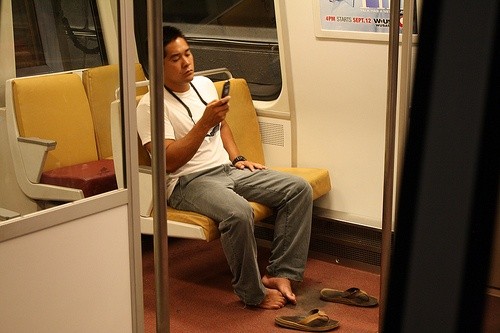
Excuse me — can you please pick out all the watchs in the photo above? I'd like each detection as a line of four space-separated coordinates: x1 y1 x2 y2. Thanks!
232 156 248 166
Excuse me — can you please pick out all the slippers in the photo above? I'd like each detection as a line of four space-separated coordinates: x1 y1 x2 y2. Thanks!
274 308 341 332
320 287 378 308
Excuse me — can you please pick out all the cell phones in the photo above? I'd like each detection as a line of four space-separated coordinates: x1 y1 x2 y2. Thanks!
221 81 230 106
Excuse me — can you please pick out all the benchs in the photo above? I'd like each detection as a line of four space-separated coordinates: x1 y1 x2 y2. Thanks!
4 62 331 242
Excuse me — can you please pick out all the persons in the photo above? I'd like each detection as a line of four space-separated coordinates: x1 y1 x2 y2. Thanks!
135 26 313 309
